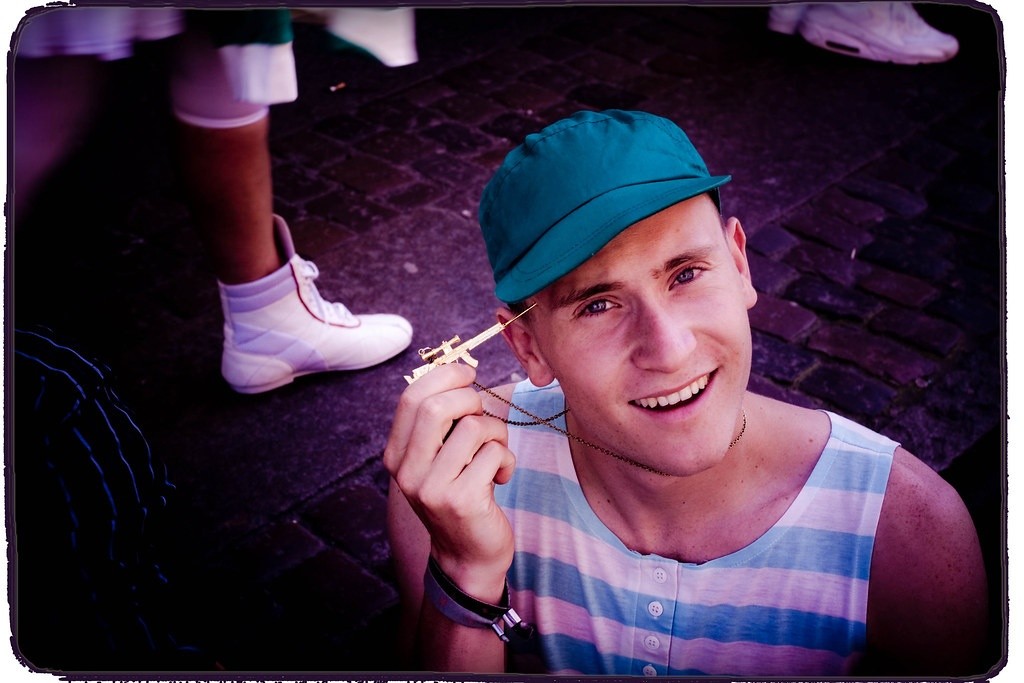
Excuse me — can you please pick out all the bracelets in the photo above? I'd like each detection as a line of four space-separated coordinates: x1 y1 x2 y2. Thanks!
423 552 537 645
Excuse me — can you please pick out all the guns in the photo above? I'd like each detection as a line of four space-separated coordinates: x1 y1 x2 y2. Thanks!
401 301 539 386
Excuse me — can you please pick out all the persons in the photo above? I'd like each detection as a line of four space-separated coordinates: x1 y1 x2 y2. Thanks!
381 111 989 676
14 8 419 393
766 4 959 64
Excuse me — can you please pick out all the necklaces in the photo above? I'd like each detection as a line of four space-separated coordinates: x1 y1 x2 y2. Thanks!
472 380 746 477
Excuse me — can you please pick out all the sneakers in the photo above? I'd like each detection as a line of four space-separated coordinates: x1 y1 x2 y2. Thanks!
798 0 959 63
767 4 804 33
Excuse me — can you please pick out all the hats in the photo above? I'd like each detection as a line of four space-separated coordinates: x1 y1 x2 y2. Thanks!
477 108 732 304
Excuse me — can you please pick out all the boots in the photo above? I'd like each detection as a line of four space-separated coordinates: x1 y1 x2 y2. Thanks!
214 212 413 394
